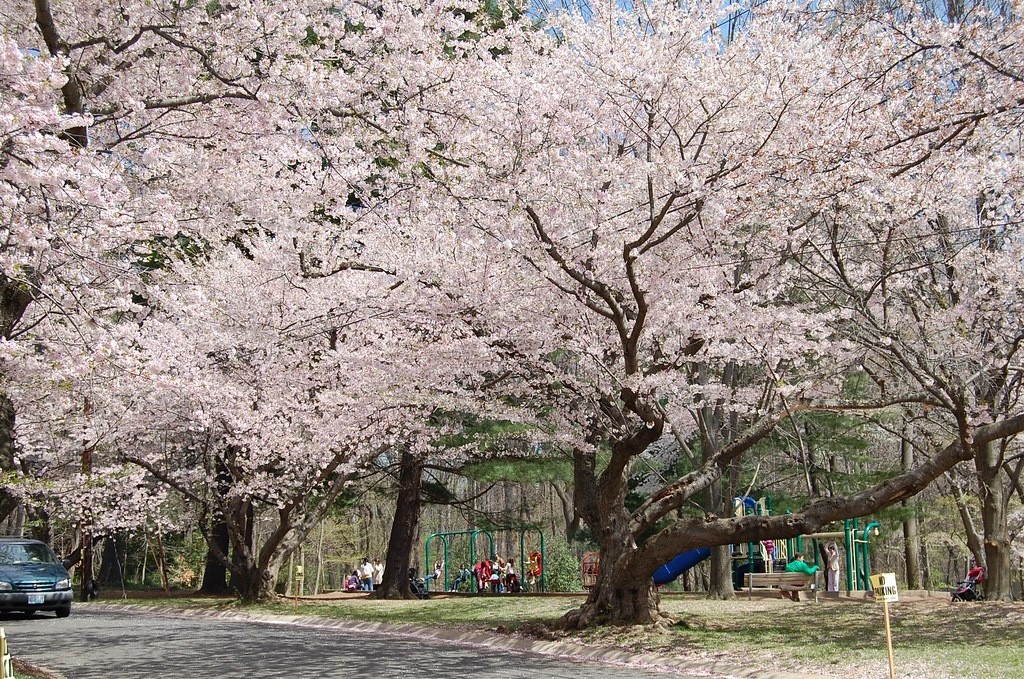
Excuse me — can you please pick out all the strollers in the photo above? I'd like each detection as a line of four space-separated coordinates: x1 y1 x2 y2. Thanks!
409 568 431 600
951 566 984 603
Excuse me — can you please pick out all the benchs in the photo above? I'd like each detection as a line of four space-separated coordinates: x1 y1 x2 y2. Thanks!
741 572 819 602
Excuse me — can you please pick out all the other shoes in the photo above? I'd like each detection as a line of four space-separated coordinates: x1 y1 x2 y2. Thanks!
530 581 535 584
501 591 504 593
520 586 523 590
507 590 511 593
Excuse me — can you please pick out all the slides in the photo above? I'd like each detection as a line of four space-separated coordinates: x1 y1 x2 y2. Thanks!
652 547 711 586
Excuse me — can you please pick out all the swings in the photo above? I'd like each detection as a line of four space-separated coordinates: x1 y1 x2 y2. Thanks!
485 531 503 583
431 535 454 579
512 531 541 575
457 534 469 581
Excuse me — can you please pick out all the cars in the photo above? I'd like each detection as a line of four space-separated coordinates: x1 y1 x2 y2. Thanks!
0 540 74 618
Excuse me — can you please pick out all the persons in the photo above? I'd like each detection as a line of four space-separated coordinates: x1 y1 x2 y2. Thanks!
762 540 778 560
421 561 444 582
779 553 820 601
451 564 470 592
822 542 840 592
345 558 385 591
474 555 538 593
